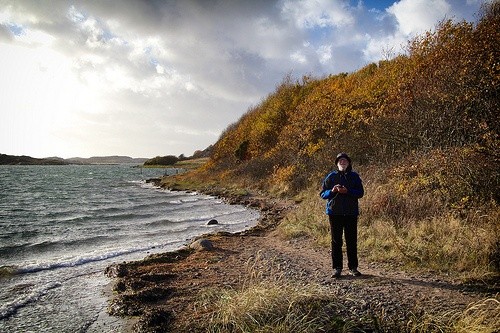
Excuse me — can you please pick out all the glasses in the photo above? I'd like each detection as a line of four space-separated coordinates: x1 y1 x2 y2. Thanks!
338 158 348 161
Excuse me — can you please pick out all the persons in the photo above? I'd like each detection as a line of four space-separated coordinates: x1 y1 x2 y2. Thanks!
318 152 365 275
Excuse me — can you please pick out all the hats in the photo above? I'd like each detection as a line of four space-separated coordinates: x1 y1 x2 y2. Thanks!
334 152 351 164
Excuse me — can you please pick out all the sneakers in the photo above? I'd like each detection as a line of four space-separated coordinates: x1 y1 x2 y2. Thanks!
330 268 342 278
348 267 361 275
